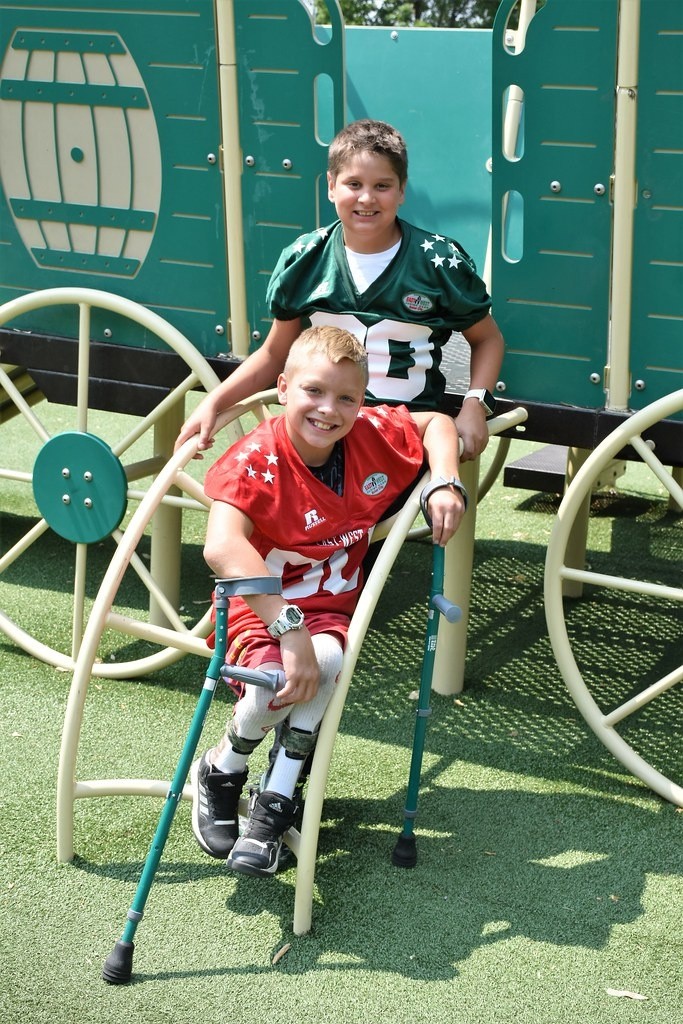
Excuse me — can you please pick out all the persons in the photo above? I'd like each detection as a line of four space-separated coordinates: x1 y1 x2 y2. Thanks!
191 325 469 878
173 119 503 581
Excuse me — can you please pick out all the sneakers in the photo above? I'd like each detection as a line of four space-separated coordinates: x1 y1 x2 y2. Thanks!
189 745 251 861
225 786 305 878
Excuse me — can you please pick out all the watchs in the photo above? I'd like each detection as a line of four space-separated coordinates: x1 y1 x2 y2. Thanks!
463 389 497 416
267 605 305 639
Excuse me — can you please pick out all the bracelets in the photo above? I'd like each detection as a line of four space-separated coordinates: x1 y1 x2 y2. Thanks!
420 476 469 530
